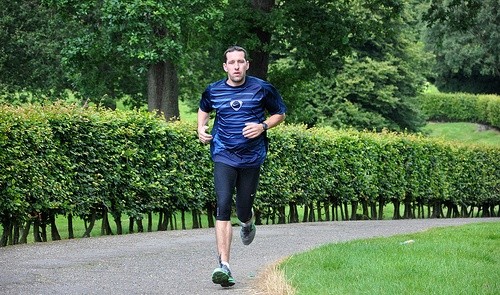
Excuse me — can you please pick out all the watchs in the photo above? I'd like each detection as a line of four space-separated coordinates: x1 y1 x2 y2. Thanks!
260 122 268 131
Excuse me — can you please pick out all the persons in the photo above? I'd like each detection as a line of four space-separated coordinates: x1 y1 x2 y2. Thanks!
196 45 287 288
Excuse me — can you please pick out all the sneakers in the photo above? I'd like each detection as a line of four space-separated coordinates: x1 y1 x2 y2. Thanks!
240 206 256 245
212 263 235 287
